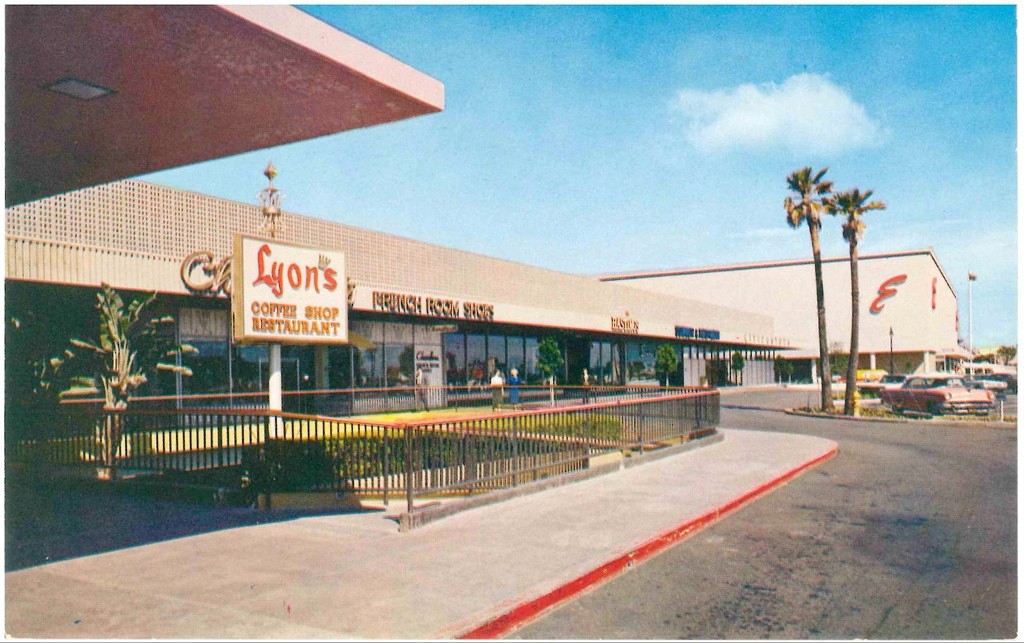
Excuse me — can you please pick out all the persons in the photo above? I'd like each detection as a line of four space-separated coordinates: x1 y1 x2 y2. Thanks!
580 368 592 405
416 368 429 413
508 368 520 409
491 370 503 412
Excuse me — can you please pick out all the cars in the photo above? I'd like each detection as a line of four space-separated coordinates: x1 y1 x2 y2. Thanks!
856 375 1009 416
638 369 656 379
